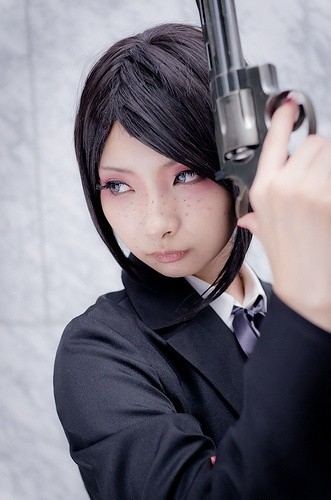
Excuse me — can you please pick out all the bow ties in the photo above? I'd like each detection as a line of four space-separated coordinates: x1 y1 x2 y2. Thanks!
231 297 270 358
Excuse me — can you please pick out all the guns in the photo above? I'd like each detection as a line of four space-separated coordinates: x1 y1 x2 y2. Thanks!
193 0 317 198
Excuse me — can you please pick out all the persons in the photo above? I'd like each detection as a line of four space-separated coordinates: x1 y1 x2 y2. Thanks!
50 18 331 500
